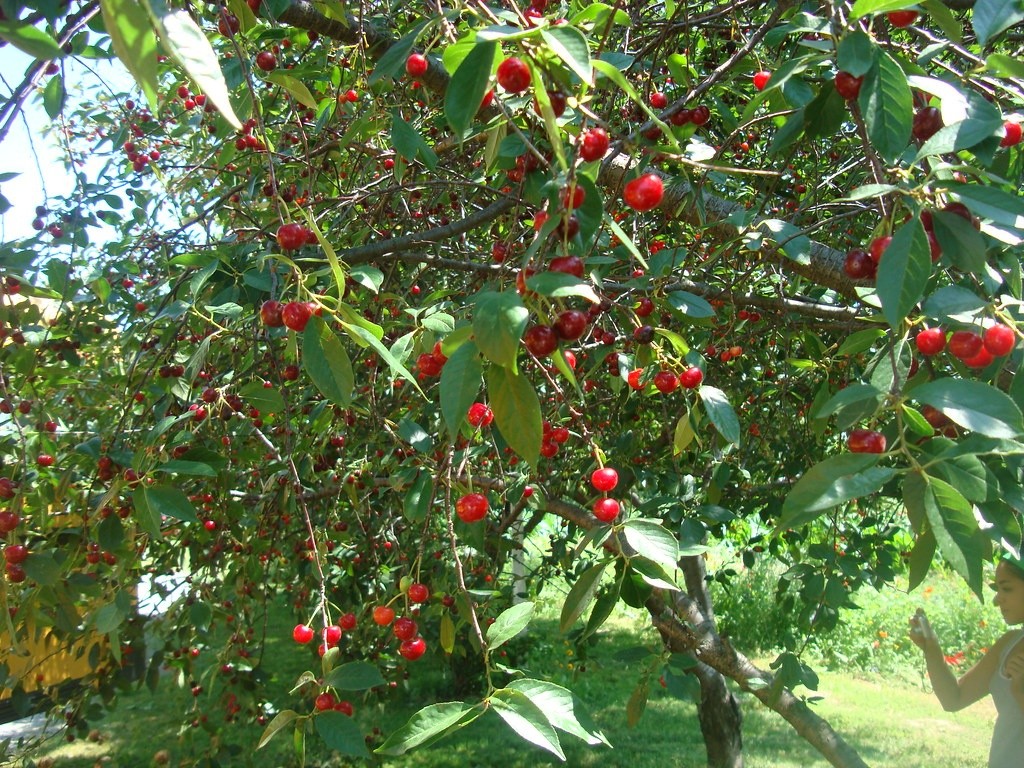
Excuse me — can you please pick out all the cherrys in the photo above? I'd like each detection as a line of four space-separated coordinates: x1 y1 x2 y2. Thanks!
0 0 1023 734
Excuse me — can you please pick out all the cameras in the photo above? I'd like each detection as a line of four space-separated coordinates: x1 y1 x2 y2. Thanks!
914 615 931 639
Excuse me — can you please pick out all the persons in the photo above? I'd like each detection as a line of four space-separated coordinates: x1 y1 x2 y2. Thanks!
907 556 1024 768
